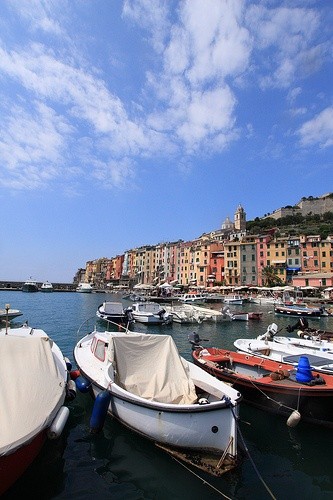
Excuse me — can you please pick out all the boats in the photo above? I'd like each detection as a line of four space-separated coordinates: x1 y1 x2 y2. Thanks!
0 305 24 321
123 286 251 306
96 301 129 322
233 323 333 376
190 330 333 425
41 281 54 292
0 303 76 460
273 299 333 319
22 280 39 292
76 282 94 293
272 330 333 354
128 302 263 324
73 310 243 475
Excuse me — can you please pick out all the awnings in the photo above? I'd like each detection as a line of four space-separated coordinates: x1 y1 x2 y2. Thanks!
272 261 286 263
287 267 300 271
170 280 177 283
153 277 158 280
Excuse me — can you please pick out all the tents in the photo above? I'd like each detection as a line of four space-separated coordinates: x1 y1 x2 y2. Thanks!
219 286 319 290
133 283 180 289
